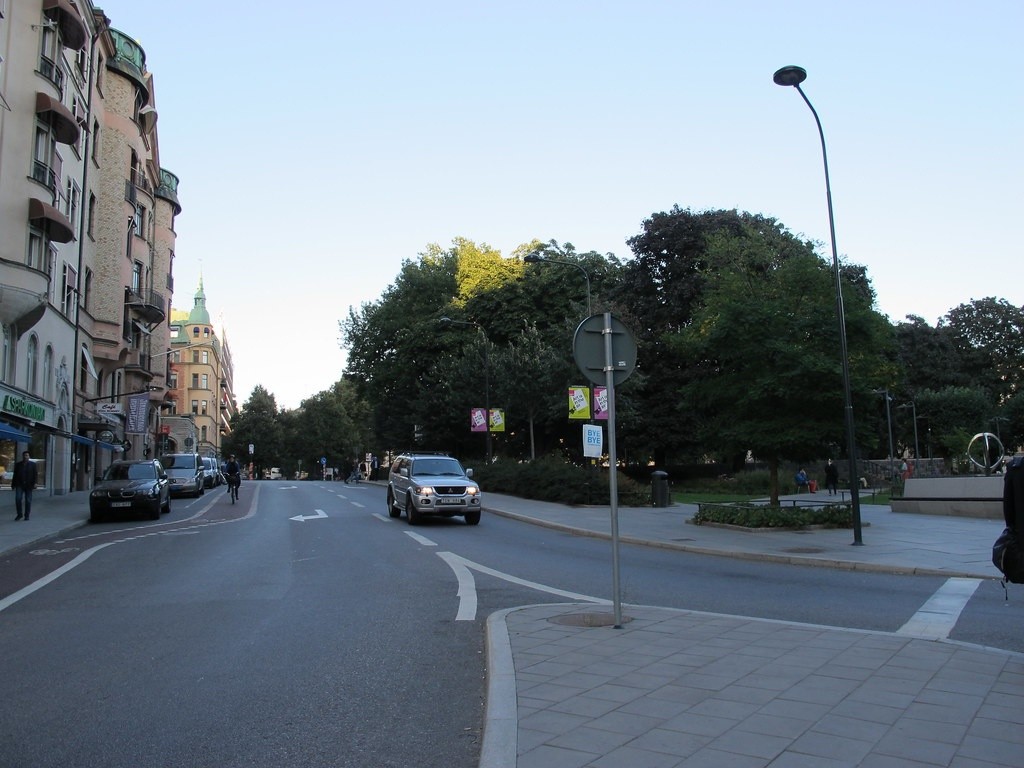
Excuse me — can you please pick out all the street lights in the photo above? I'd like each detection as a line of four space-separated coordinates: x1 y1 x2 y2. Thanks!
438 317 493 460
868 384 895 487
772 66 865 545
896 400 919 479
524 253 592 316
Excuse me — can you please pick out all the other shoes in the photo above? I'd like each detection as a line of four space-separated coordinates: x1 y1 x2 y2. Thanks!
236 496 239 500
15 514 23 521
228 489 230 493
24 513 29 521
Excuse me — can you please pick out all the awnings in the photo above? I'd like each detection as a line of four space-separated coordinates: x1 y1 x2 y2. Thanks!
134 322 151 335
37 92 80 144
0 423 32 443
82 346 98 380
36 421 114 450
43 0 86 50
140 105 158 134
29 198 74 243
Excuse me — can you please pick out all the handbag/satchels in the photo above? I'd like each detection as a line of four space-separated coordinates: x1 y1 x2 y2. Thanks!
990 526 1024 583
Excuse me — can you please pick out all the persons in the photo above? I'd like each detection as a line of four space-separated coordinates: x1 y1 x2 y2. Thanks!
360 461 366 480
224 455 241 499
901 458 909 479
346 458 360 484
370 457 380 480
11 451 38 520
796 468 817 493
825 459 839 496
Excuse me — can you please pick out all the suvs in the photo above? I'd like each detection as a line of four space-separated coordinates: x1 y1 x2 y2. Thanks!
159 452 205 498
386 450 481 526
199 456 227 489
89 459 173 521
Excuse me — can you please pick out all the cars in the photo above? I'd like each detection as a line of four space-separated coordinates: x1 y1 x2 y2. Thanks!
991 456 1024 592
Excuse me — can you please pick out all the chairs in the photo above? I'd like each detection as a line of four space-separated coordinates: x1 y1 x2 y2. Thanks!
793 475 810 495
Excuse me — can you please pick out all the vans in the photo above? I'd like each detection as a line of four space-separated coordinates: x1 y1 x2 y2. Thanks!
264 467 282 480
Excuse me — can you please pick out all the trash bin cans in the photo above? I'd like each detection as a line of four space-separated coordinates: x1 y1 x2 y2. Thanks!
652 471 668 507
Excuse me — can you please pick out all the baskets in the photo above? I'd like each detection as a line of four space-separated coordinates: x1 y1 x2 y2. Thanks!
228 476 238 484
